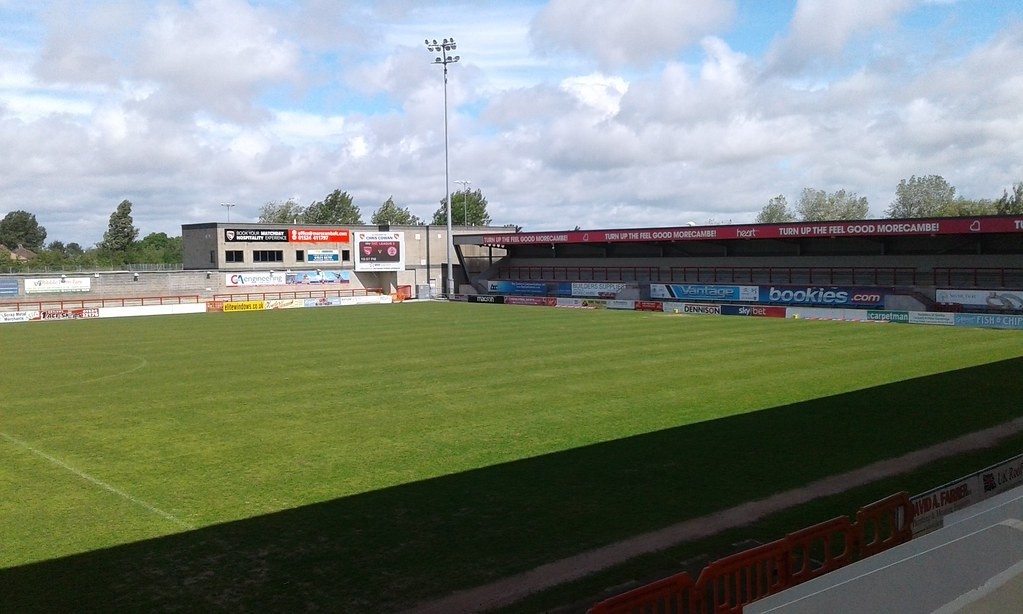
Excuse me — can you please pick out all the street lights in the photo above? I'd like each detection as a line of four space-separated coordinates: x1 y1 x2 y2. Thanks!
454 179 471 226
221 203 235 223
425 37 468 297
